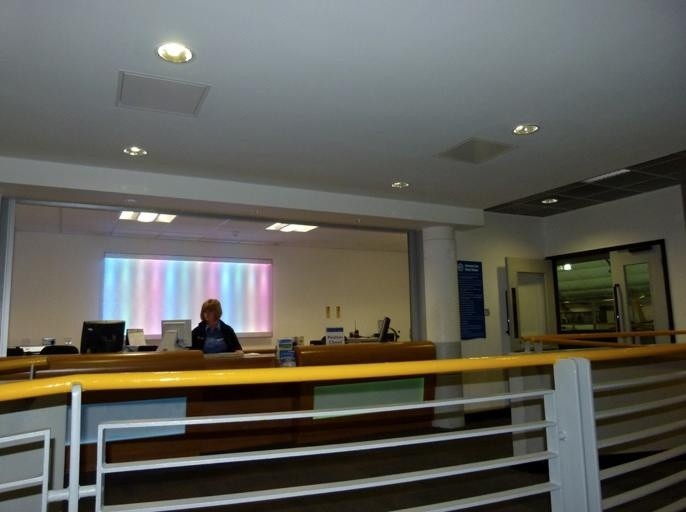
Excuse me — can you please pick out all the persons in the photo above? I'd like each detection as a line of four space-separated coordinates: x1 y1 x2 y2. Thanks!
184 298 243 352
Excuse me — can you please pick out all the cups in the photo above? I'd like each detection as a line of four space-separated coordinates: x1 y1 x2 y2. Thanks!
63 336 73 345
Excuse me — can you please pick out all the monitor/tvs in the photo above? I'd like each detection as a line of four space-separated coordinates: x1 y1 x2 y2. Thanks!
379 317 390 342
79 320 125 354
156 319 192 351
